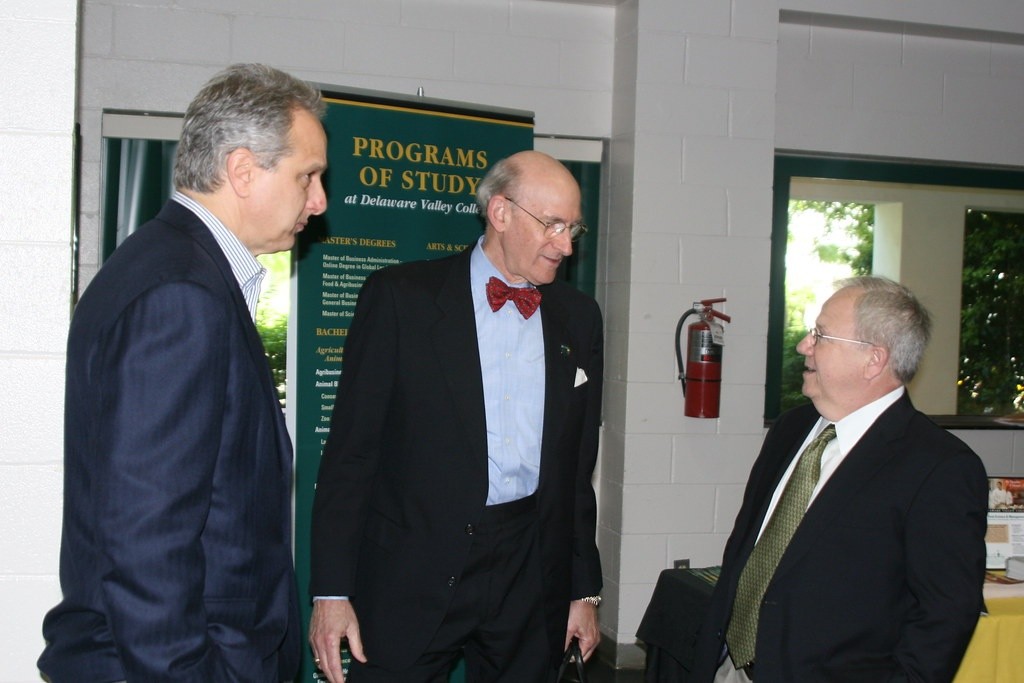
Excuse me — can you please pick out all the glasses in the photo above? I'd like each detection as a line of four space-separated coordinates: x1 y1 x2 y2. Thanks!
505 197 589 242
810 326 877 348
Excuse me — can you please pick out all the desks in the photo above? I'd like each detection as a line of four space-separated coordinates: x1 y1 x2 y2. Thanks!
633 566 1024 683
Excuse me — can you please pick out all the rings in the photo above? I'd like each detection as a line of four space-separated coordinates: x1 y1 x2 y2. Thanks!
314 658 320 665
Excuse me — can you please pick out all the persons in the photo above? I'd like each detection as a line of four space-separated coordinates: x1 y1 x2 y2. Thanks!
699 277 989 683
37 63 328 683
989 482 1013 509
309 150 602 683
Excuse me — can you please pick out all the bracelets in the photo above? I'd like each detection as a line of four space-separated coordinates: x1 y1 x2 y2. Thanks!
581 596 601 605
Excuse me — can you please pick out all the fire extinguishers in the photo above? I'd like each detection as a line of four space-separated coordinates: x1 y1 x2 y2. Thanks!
675 298 730 419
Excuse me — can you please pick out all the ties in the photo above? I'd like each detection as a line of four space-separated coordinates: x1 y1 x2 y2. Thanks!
725 423 837 669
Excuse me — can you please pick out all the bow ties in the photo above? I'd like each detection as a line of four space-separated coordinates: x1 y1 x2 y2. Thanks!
486 276 543 319
